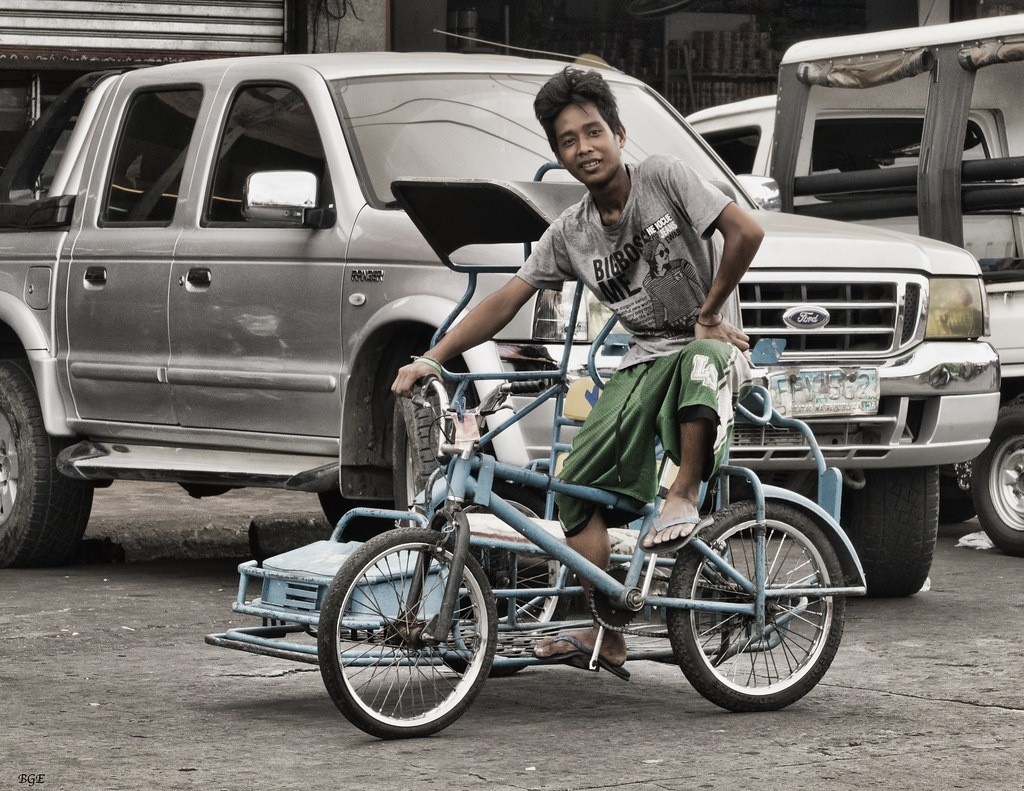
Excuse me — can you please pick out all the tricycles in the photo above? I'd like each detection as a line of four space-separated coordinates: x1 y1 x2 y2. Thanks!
203 161 870 739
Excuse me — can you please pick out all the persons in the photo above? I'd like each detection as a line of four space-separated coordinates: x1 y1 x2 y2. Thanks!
391 66 765 669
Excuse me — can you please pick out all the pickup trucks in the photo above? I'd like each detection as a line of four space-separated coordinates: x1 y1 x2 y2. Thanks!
683 14 1024 563
0 50 1000 606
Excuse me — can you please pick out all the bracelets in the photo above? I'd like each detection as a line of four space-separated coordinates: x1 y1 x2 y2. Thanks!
415 356 442 374
696 314 723 327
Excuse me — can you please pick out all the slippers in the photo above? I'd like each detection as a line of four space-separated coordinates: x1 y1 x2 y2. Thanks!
533 634 631 682
639 514 714 553
